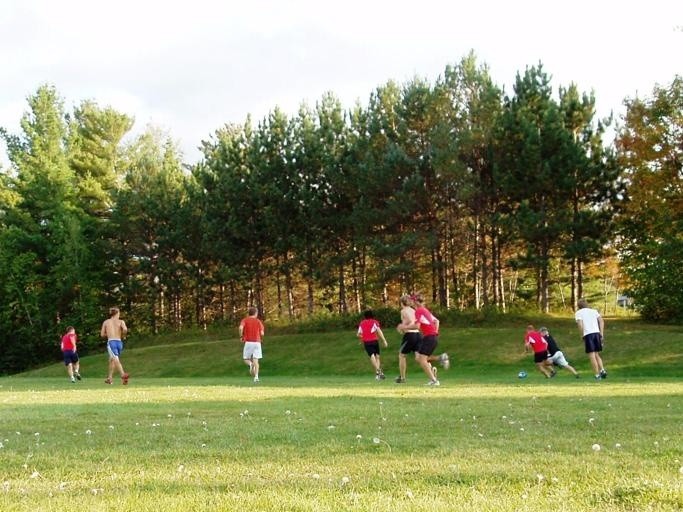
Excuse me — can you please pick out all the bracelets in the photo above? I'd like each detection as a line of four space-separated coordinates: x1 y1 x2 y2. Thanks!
240 335 243 338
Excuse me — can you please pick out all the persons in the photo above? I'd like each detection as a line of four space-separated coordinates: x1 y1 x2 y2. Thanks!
99 306 130 385
356 309 388 380
397 294 449 387
534 325 580 381
395 294 439 386
238 306 266 383
523 323 554 380
59 325 81 383
574 299 608 382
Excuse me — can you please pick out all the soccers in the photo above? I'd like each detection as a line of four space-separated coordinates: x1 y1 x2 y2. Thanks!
518 371 527 378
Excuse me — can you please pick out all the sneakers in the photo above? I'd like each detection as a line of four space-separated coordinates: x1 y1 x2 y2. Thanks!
594 376 602 381
122 373 128 385
550 370 556 376
431 366 438 377
394 377 406 384
601 371 607 379
73 371 82 381
104 378 113 384
425 381 440 386
441 353 450 370
375 371 386 381
250 362 254 376
71 377 77 382
254 379 260 382
575 374 579 378
